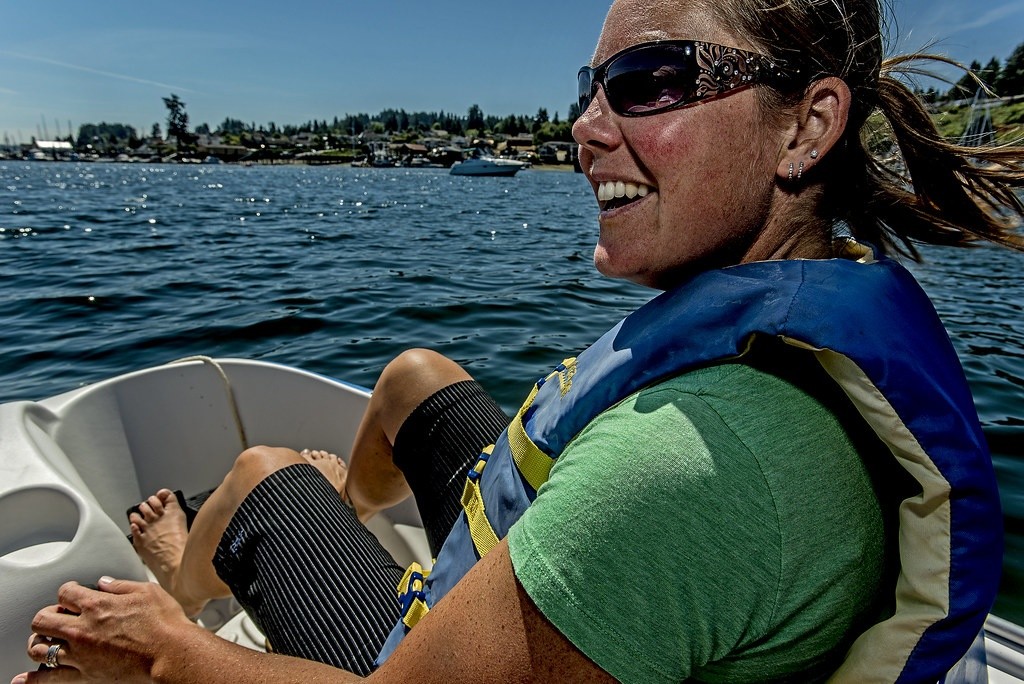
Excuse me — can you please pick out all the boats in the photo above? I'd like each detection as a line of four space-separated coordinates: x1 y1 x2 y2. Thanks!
449 155 525 176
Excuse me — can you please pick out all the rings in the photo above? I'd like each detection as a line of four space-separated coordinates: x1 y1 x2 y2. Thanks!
46 641 61 669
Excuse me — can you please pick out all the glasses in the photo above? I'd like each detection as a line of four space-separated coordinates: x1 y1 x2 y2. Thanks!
577 39 788 118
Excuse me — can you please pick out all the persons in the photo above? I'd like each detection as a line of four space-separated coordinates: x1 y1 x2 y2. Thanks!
12 1 1024 684
